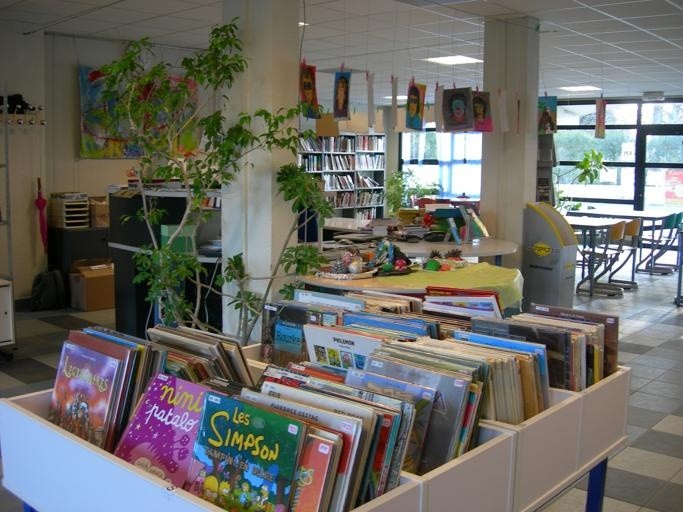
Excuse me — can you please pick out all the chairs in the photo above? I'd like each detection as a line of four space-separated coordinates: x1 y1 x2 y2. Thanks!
565 206 683 296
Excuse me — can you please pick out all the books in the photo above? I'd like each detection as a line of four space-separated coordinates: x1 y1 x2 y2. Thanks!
45 283 622 511
299 133 481 242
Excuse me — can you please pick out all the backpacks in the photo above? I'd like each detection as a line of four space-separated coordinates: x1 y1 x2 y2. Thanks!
29 269 66 311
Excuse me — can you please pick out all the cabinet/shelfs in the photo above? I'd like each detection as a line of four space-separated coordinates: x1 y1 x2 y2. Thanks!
49 196 91 231
297 131 386 242
47 226 112 311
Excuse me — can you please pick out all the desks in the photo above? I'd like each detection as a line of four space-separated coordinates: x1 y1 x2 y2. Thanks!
1 340 633 511
106 242 222 341
297 259 524 314
378 237 518 268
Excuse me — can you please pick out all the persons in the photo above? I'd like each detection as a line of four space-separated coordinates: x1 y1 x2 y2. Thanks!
471 96 492 131
405 86 422 130
333 77 349 117
538 105 555 133
444 92 470 131
301 70 320 119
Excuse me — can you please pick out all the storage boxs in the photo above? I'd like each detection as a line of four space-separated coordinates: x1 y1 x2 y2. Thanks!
89 195 109 228
69 258 115 311
